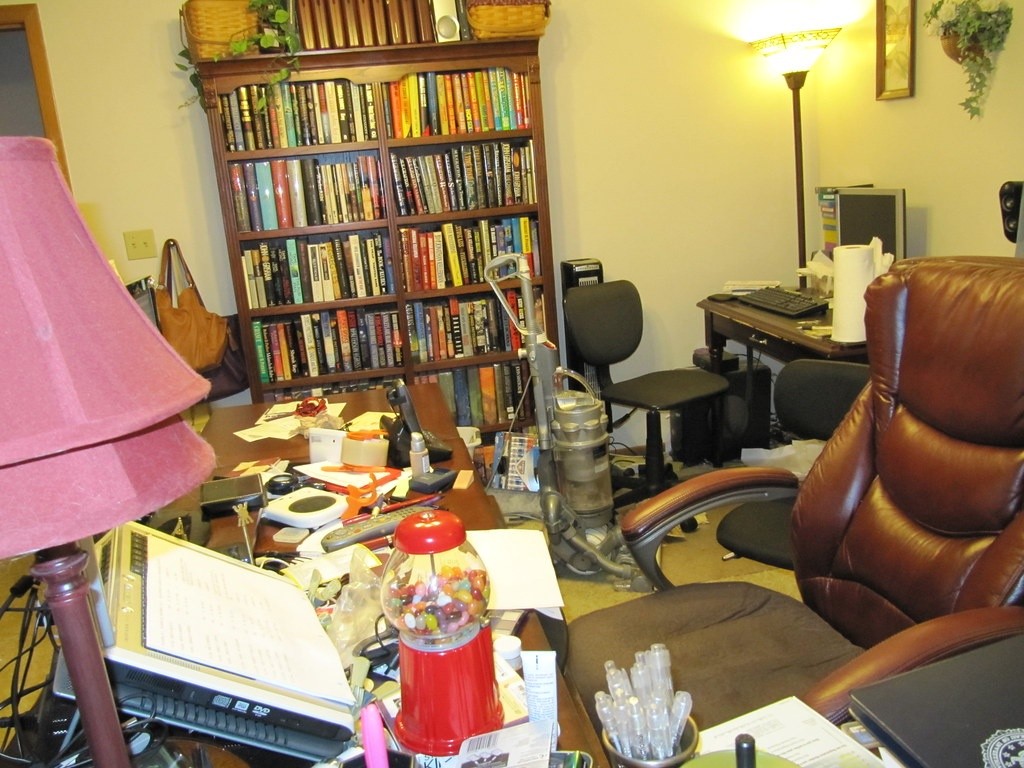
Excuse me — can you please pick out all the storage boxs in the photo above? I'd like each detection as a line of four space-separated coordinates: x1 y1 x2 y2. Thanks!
467 1 550 39
178 0 260 60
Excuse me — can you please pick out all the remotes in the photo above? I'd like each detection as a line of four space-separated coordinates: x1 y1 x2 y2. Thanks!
321 506 435 554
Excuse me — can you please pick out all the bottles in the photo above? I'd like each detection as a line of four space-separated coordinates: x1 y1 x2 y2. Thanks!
409 432 429 476
381 512 504 757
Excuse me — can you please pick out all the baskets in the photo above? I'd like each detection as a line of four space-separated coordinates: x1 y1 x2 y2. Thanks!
465 0 552 40
179 0 261 64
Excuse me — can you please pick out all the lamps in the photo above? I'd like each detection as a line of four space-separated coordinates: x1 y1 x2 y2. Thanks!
749 19 842 293
1 133 214 766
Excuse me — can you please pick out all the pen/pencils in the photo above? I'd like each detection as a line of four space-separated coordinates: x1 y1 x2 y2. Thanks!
595 643 693 761
379 495 435 511
420 496 444 507
797 326 832 330
343 516 372 524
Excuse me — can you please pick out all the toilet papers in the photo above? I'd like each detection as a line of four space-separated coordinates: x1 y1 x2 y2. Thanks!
830 243 877 343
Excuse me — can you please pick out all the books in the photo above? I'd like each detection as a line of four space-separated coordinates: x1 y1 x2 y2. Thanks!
406 291 543 363
391 139 537 215
415 360 530 426
383 67 531 138
228 156 387 233
219 79 378 152
400 216 542 291
240 232 394 309
814 183 870 252
252 310 404 384
263 376 404 403
297 0 432 49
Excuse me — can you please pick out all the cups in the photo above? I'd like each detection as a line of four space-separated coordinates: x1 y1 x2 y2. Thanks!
602 716 699 768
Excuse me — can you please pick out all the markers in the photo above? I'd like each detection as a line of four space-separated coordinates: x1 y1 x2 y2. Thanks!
358 535 396 550
736 734 755 768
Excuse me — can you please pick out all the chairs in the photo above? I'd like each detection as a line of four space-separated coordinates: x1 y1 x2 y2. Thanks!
717 359 874 571
564 252 1024 767
564 281 728 532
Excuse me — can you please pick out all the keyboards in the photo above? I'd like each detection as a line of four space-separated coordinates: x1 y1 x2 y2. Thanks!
735 286 829 319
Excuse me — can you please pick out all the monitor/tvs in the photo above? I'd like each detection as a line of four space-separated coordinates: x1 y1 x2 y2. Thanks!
836 187 906 266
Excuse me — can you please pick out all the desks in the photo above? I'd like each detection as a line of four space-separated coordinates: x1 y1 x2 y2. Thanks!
151 385 608 768
698 300 868 359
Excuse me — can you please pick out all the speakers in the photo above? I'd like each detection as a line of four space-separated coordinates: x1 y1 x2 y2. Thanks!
999 180 1024 243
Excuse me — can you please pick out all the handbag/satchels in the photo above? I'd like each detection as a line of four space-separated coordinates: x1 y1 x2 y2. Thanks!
149 238 250 403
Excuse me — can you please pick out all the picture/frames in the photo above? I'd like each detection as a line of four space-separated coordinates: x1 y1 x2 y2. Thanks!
876 0 916 100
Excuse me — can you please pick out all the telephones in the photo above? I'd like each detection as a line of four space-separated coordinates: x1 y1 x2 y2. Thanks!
386 379 452 467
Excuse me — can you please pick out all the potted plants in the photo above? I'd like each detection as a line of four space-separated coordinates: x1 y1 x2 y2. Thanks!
924 0 1012 116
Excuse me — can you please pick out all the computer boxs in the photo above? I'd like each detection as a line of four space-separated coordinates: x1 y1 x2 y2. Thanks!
670 358 772 466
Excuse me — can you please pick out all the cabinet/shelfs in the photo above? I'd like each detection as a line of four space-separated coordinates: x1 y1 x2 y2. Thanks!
196 33 562 436
